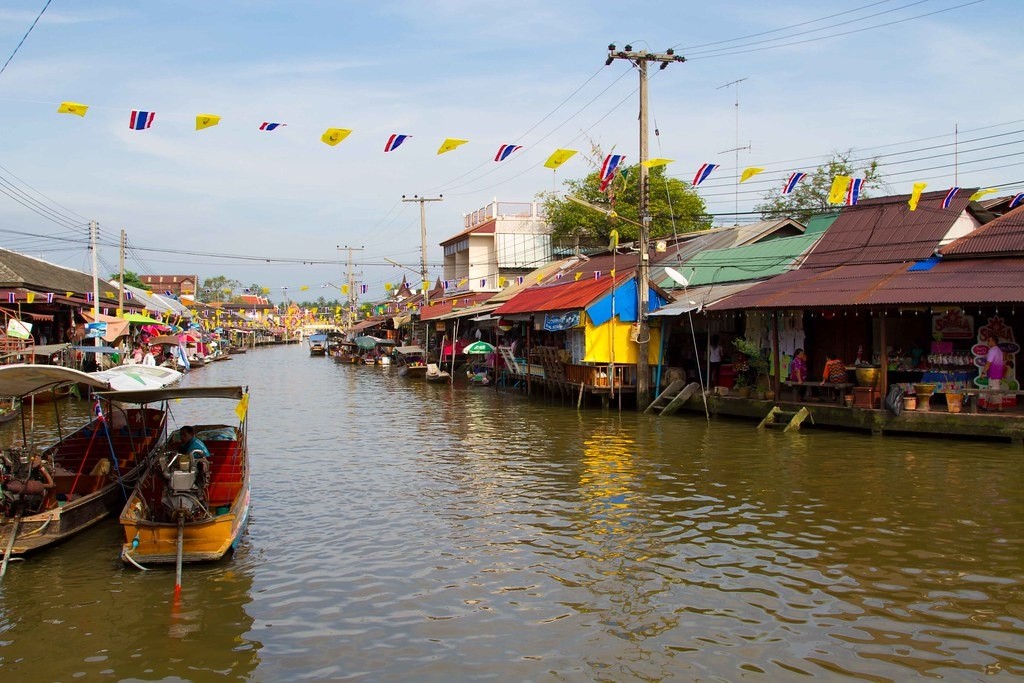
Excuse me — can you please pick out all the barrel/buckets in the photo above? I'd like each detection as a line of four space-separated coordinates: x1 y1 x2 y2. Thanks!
855 365 882 387
945 394 963 412
903 396 917 410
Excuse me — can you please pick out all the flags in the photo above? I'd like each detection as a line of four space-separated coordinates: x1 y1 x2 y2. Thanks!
93 401 106 422
179 342 190 368
234 393 249 420
58 102 352 147
384 133 719 193
739 166 1024 211
8 271 615 331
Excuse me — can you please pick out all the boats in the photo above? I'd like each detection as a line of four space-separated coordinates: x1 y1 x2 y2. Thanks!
319 331 451 382
467 371 492 386
148 326 251 375
0 362 251 564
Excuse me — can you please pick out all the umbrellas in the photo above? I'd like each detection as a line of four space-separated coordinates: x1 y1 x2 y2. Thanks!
356 337 377 350
462 340 496 368
115 311 164 325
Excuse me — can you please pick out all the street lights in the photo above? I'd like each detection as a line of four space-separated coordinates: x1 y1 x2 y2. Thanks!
384 257 428 307
563 194 651 412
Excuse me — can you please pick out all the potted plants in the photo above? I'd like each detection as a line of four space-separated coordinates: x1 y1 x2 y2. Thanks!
932 331 964 413
856 364 882 387
731 337 775 401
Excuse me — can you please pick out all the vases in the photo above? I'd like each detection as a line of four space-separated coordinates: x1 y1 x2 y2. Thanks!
903 397 916 411
913 383 936 411
844 394 853 407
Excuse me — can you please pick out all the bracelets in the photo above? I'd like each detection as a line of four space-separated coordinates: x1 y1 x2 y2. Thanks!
982 372 986 375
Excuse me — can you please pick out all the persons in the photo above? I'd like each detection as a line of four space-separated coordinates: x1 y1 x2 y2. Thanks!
790 348 807 402
180 425 210 458
820 351 849 399
980 334 1004 413
704 335 723 390
131 344 144 364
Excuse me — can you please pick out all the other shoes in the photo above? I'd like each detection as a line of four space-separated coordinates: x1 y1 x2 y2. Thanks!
979 406 987 412
991 408 1003 413
